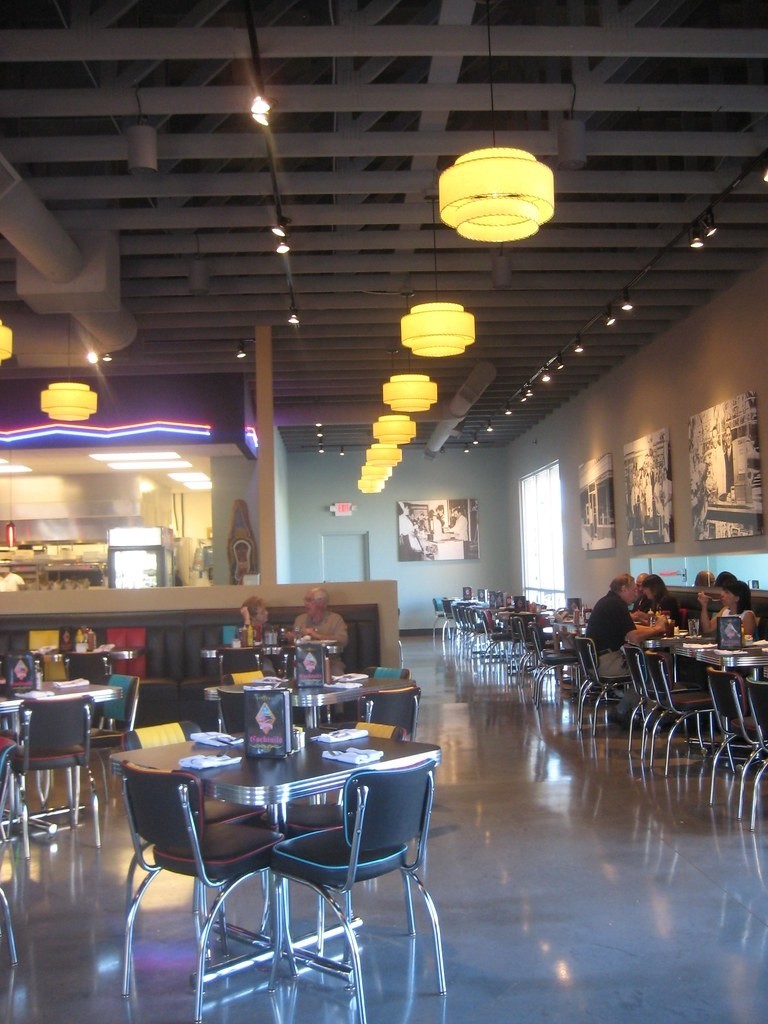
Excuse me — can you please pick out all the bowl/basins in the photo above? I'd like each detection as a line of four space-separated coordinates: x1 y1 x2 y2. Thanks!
243 575 260 587
39 580 91 590
745 634 753 646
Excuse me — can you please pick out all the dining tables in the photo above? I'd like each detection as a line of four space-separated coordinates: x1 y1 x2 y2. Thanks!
1 648 138 676
543 625 587 685
110 721 443 1011
641 635 717 725
203 677 414 733
695 650 768 769
201 645 345 685
671 641 768 749
0 681 123 843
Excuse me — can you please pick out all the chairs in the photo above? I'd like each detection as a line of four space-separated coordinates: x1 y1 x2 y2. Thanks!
0 597 768 1024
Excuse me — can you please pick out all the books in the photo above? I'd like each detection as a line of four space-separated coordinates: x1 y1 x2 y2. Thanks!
243 689 294 759
296 644 324 687
462 587 582 615
58 626 87 652
5 651 36 697
717 616 742 650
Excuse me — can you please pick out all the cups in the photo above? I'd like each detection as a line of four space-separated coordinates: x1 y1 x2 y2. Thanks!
688 619 699 636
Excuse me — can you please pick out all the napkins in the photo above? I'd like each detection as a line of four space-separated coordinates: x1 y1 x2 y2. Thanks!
324 682 363 689
94 644 116 651
714 649 749 655
322 746 385 765
252 676 288 684
51 678 90 688
242 684 293 694
189 732 244 747
331 673 369 681
762 648 768 652
14 691 54 700
41 645 55 653
179 754 242 769
310 729 369 743
753 639 768 646
681 642 718 649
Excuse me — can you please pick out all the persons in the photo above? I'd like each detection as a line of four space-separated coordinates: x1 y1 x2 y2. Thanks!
0 559 25 592
710 426 760 501
714 571 737 588
626 461 673 546
586 573 668 723
398 500 469 562
630 573 649 613
284 587 349 728
694 571 715 587
629 574 682 630
236 595 281 677
697 580 756 643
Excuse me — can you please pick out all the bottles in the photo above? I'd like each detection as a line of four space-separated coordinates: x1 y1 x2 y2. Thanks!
573 604 591 625
192 546 213 572
646 610 673 636
75 626 97 652
264 625 277 646
741 621 745 647
35 668 43 689
239 623 254 647
293 729 301 749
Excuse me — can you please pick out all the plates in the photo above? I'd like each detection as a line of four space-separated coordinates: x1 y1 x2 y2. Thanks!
674 633 688 638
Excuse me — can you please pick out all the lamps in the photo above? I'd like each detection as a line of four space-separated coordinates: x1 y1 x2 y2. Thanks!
559 80 586 169
128 86 158 171
400 197 476 355
465 150 768 453
438 0 555 243
40 316 98 420
383 349 438 411
0 320 13 364
104 91 345 456
358 411 417 493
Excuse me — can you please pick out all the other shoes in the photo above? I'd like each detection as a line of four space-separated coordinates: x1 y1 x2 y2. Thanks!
632 715 639 730
608 708 629 730
